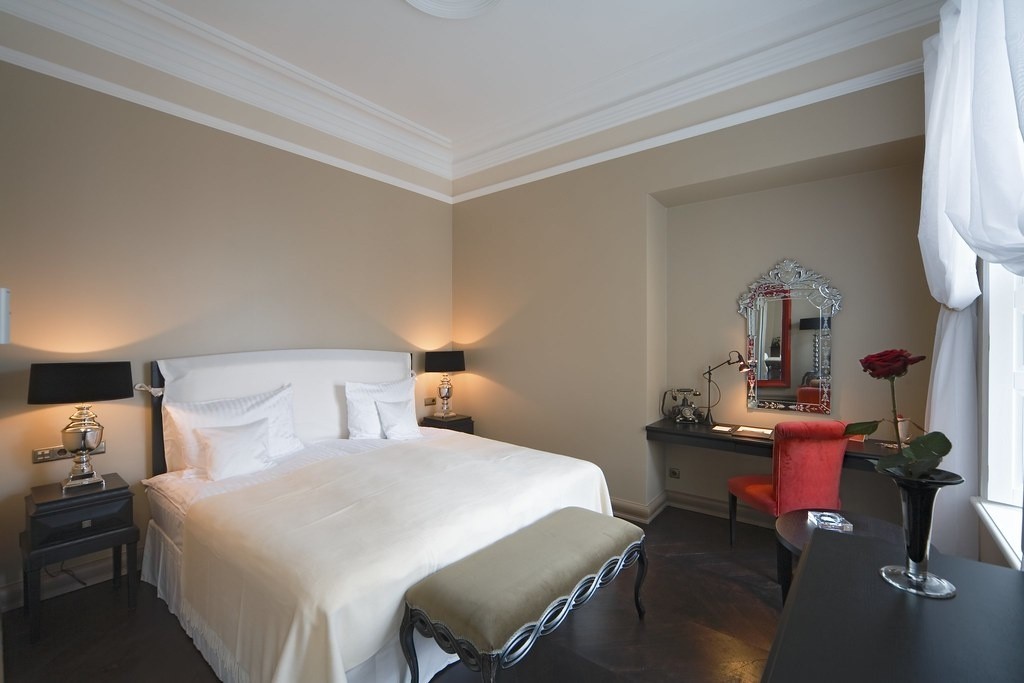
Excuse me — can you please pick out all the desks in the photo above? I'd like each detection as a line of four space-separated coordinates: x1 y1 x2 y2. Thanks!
762 528 1024 683
765 356 782 380
645 413 911 475
776 507 942 608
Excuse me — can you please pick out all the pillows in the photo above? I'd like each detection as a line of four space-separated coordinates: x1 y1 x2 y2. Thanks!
344 371 416 442
165 383 305 480
375 400 423 439
193 416 279 481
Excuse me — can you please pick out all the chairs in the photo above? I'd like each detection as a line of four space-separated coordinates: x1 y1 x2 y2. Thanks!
726 419 851 550
796 387 830 413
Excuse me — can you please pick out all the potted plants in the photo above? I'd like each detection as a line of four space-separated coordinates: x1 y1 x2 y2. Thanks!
770 337 781 357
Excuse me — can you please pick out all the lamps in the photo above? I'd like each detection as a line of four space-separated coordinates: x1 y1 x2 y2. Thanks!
701 349 751 427
28 361 135 496
424 350 467 419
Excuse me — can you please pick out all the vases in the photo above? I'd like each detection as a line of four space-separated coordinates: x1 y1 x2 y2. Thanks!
881 466 967 600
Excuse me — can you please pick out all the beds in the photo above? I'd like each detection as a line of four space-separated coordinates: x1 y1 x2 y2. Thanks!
143 346 613 683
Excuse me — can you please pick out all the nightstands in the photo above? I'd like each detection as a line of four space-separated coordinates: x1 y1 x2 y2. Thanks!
18 472 142 644
419 413 474 436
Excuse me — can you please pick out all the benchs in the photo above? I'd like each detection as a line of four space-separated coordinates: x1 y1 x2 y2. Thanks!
398 505 649 683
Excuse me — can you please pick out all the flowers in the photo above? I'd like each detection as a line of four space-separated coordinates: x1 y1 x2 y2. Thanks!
843 348 953 477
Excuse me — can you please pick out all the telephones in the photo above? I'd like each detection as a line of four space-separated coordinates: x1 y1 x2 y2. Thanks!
670 389 701 423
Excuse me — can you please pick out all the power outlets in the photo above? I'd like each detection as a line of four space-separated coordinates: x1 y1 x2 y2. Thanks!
425 397 436 406
32 440 106 464
669 467 681 479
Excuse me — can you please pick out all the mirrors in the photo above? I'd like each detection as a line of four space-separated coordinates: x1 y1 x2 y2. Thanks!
739 259 841 417
755 286 793 388
